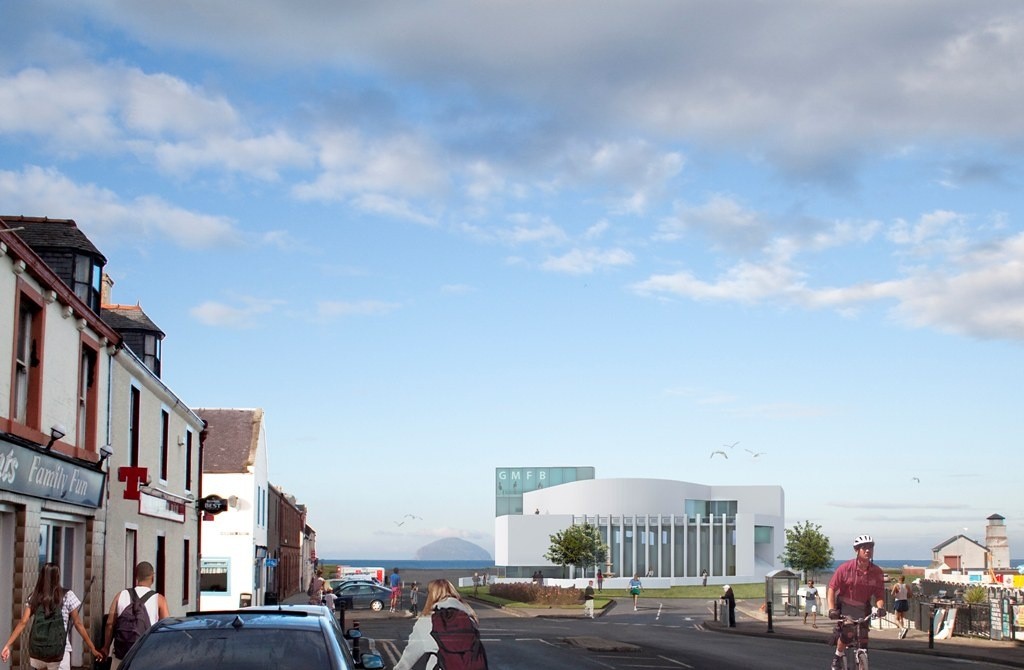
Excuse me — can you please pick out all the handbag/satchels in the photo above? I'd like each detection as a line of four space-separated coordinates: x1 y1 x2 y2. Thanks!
307 584 313 596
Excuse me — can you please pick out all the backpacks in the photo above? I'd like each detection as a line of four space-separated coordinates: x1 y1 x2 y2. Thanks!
428 606 488 670
29 589 69 663
114 588 156 660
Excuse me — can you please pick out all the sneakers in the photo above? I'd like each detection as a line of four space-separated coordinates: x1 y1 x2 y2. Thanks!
832 654 843 670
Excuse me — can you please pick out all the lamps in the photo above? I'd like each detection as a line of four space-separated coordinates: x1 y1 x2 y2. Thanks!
45 424 66 451
140 475 151 486
95 443 114 472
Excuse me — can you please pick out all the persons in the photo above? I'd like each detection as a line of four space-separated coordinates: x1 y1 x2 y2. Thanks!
626 574 644 611
646 567 654 577
584 580 595 618
828 534 885 669
703 570 707 586
891 576 912 639
532 571 543 585
2 563 103 670
802 580 821 628
409 583 419 618
311 569 337 614
473 572 480 593
393 578 479 670
597 569 603 593
720 585 736 626
102 561 169 670
390 568 400 612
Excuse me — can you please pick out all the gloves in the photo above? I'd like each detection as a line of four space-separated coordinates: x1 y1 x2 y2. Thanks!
877 609 886 618
829 609 842 619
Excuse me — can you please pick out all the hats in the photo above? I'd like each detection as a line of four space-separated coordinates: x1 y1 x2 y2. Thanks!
723 585 730 589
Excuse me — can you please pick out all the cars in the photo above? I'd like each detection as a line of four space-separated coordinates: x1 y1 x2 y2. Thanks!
234 602 363 661
326 575 395 614
111 609 386 670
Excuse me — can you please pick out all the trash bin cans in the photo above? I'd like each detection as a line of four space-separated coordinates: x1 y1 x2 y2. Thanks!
265 592 277 604
239 593 252 608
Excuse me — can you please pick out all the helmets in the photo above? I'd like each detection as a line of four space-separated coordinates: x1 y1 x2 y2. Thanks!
853 535 874 549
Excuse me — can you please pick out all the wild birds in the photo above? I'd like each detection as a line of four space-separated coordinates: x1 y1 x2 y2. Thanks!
710 440 769 460
393 513 424 527
911 477 920 484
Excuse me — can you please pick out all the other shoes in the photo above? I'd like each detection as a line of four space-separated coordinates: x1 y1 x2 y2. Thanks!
902 629 908 639
803 620 806 625
813 625 817 629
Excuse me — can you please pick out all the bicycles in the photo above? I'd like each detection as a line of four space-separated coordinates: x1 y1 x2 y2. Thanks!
831 610 880 670
